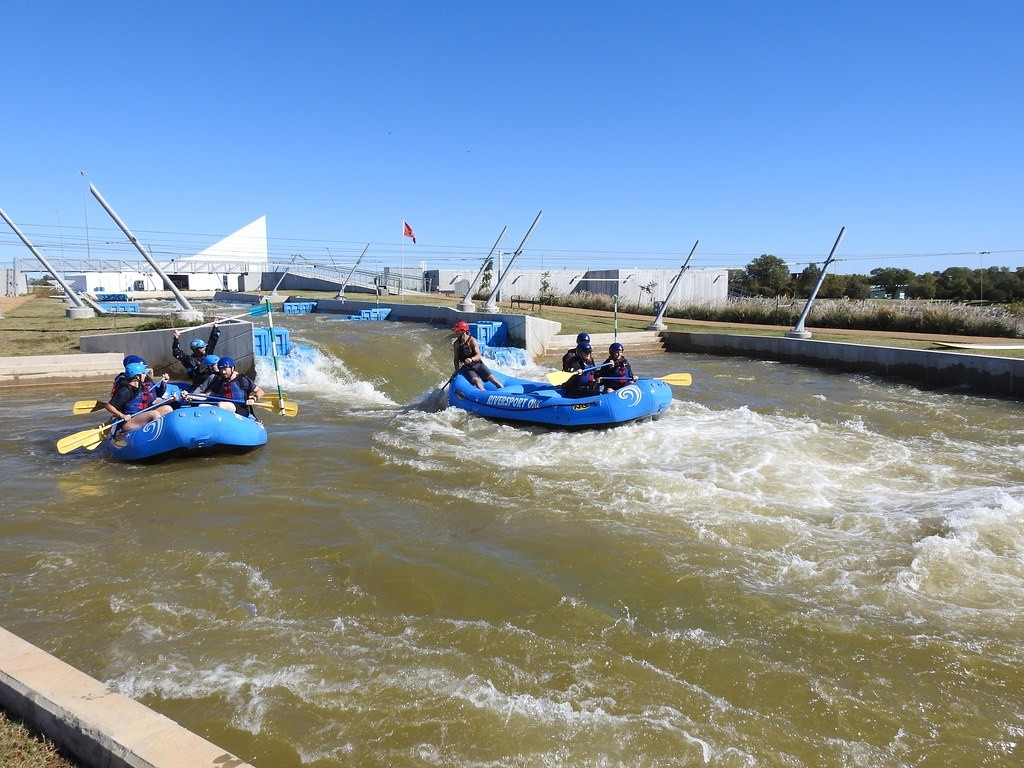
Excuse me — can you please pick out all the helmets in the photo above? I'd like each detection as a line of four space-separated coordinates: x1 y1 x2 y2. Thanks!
577 332 590 342
190 339 209 350
217 356 235 369
452 321 470 333
127 356 146 366
609 343 623 354
125 364 144 379
577 343 592 354
204 354 221 366
123 355 138 365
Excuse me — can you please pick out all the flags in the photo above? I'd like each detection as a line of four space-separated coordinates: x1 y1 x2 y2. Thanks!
402 221 416 243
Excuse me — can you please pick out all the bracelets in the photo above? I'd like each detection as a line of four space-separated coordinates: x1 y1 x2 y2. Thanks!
471 357 473 362
254 395 258 401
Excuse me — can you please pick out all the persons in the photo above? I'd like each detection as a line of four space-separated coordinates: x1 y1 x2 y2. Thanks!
562 333 638 400
172 318 220 393
105 363 178 431
451 321 503 391
181 354 220 398
184 357 264 418
111 355 170 399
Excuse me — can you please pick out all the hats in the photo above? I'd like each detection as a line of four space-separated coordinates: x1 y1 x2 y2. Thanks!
584 349 592 353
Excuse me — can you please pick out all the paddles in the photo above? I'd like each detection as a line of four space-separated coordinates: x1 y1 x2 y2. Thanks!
178 304 274 334
250 392 288 401
72 400 107 415
599 372 693 387
56 395 175 455
546 360 613 387
181 393 299 418
82 379 163 451
428 363 465 404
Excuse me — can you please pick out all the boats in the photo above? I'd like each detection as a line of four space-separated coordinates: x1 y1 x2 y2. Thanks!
446 368 671 427
106 382 268 465
